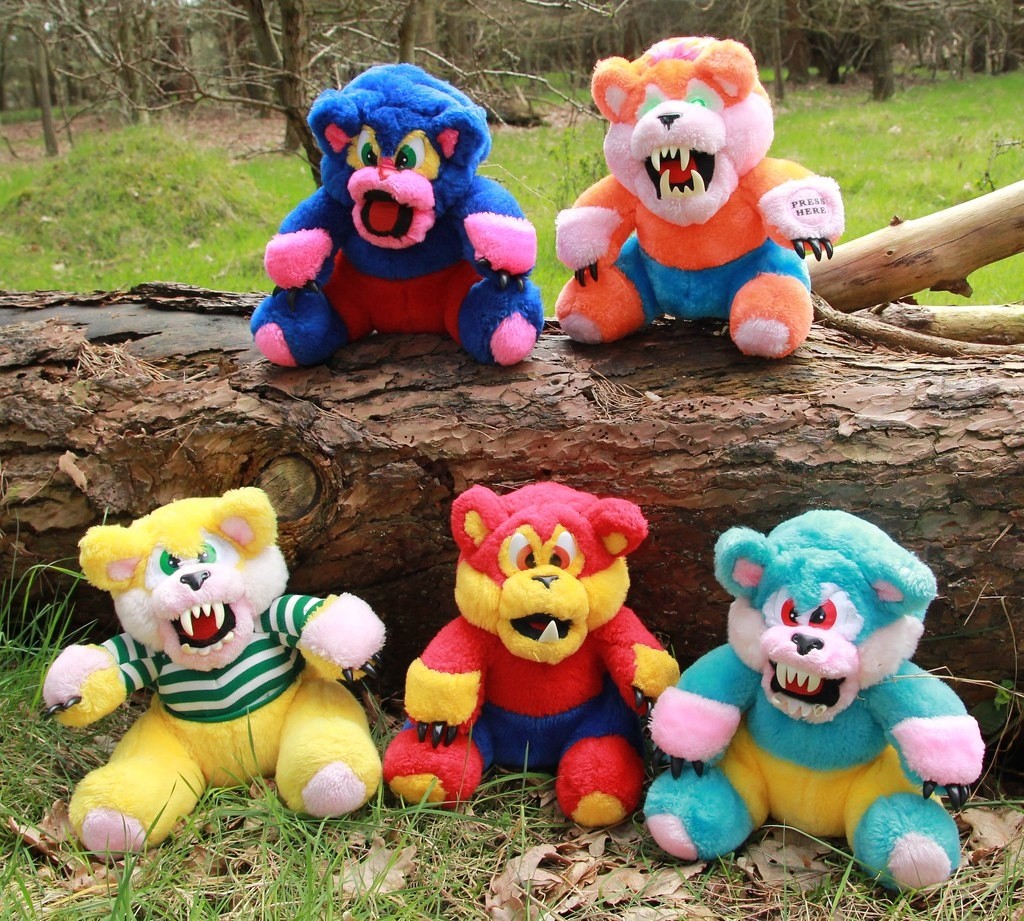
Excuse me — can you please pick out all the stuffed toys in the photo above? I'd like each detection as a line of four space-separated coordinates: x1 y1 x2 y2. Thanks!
643 508 985 893
249 63 545 366
555 37 846 358
382 483 681 826
43 488 387 865
